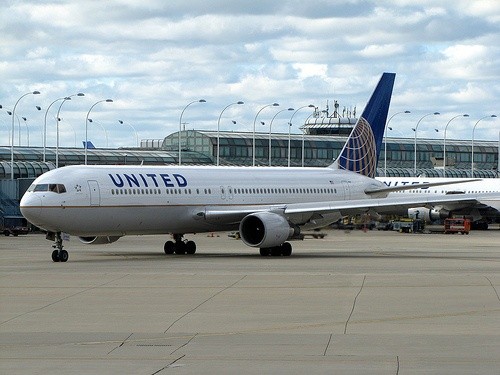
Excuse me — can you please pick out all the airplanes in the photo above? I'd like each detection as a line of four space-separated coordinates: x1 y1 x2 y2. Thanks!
20 72 500 262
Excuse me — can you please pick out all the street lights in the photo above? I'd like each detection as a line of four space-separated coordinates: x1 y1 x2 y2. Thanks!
443 114 470 177
43 97 72 162
11 90 41 180
253 103 280 166
268 107 295 166
384 110 412 177
56 93 85 168
414 112 441 176
84 99 113 165
178 99 207 166
6 110 139 148
216 101 245 166
288 104 316 167
472 115 498 177
